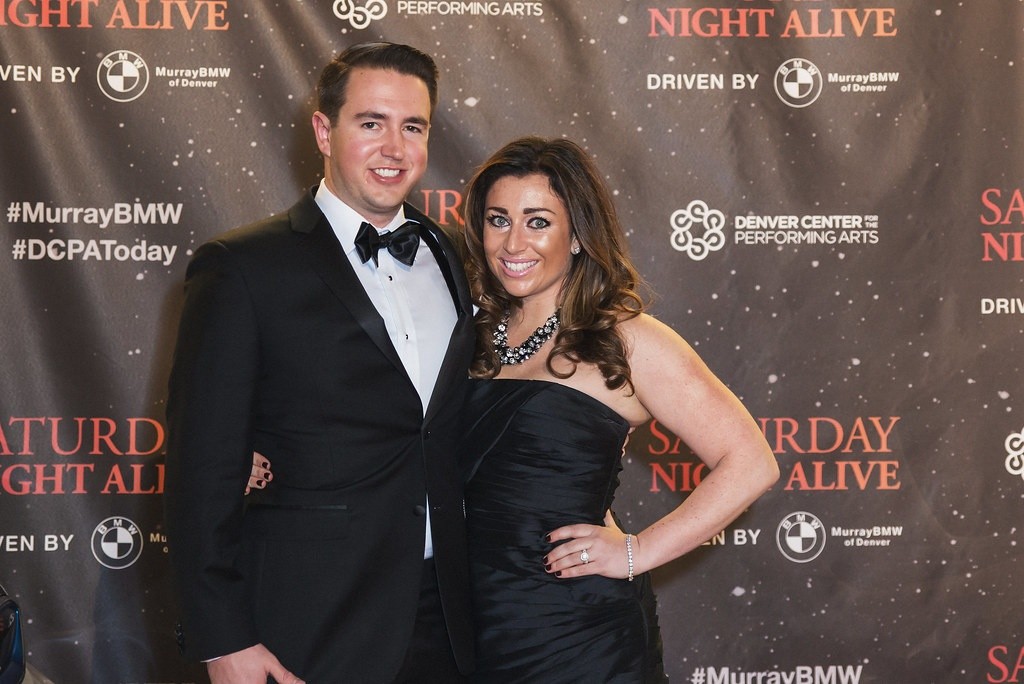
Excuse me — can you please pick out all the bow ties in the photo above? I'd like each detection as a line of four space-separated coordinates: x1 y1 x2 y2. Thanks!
354 220 422 267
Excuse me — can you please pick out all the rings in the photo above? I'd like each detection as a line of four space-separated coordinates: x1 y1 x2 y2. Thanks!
580 549 589 564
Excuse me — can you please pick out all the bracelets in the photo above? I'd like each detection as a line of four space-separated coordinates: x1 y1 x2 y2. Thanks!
626 533 635 582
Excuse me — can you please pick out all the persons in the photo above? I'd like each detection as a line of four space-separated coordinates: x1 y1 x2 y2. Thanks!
162 41 635 684
243 138 780 684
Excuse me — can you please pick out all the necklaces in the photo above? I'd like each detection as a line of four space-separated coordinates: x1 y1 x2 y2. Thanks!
491 303 573 366
